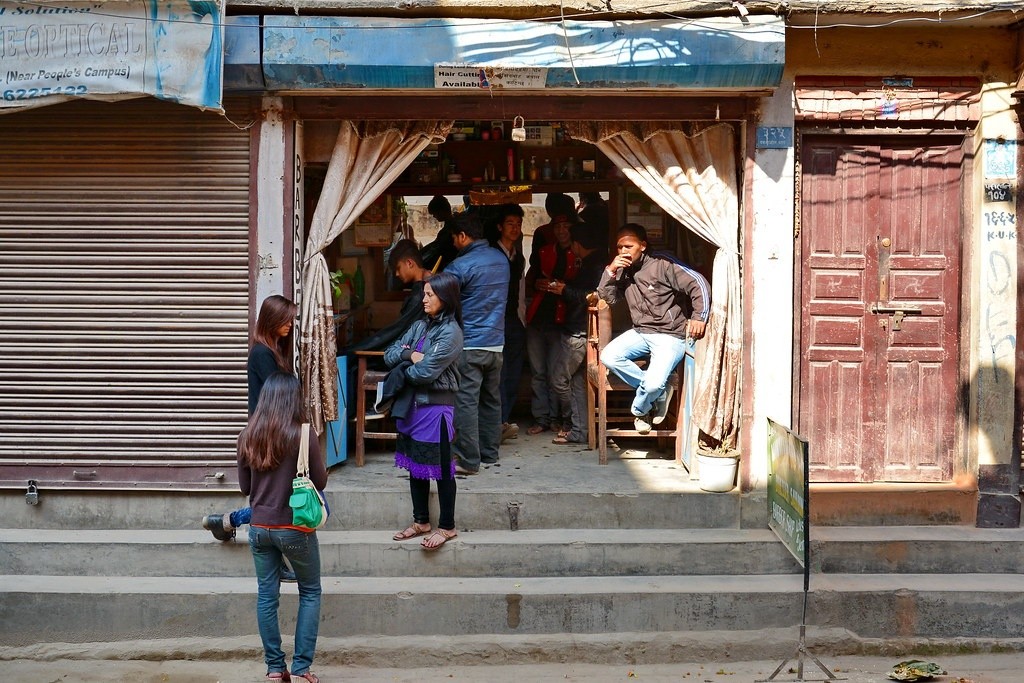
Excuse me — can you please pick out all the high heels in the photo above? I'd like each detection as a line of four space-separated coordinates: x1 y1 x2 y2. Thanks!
267 670 290 683
290 671 319 683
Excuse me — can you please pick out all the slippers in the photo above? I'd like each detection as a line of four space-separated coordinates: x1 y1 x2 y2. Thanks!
420 530 459 551
394 524 432 541
558 429 567 436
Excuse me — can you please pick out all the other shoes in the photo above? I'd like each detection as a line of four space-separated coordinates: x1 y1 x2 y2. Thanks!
552 437 569 444
454 465 476 476
504 422 519 439
480 460 501 468
552 423 558 431
527 425 543 435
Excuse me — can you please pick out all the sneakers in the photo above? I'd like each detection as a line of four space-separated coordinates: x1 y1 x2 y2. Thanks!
652 384 674 425
635 414 653 433
280 557 298 583
203 511 236 541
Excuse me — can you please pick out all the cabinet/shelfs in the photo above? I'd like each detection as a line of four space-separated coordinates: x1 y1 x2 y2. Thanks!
390 120 616 188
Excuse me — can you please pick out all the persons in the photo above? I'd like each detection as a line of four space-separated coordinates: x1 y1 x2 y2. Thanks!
384 273 463 552
597 223 713 433
202 295 305 582
236 372 329 683
344 192 608 476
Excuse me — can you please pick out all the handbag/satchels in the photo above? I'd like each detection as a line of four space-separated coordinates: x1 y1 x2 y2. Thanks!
289 423 330 530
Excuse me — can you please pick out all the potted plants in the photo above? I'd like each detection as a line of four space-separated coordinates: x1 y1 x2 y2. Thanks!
695 433 741 492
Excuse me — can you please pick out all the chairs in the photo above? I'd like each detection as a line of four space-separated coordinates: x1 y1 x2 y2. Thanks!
586 291 681 464
355 349 397 465
